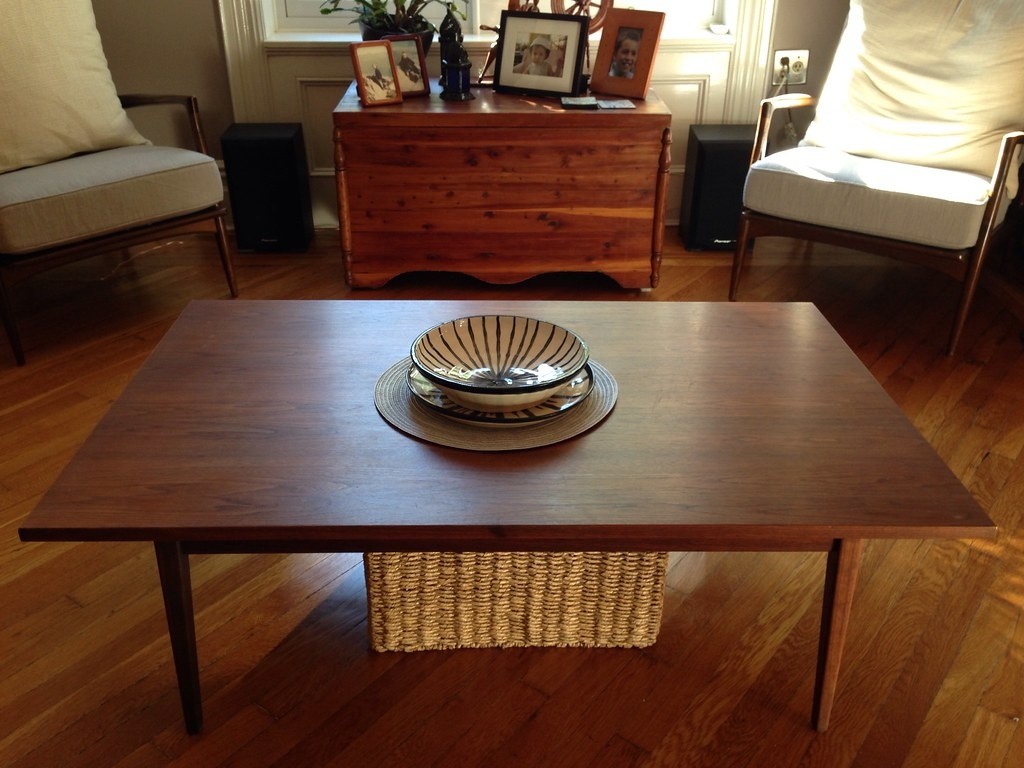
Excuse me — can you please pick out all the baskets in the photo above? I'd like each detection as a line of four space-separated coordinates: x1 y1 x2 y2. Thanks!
363 550 669 653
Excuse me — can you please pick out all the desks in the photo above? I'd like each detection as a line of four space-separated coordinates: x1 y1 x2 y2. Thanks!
14 297 1001 738
331 78 672 292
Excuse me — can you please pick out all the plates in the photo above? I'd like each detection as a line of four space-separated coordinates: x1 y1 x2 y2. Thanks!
405 362 596 428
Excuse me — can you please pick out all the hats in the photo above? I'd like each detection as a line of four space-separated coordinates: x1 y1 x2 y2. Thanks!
529 37 551 51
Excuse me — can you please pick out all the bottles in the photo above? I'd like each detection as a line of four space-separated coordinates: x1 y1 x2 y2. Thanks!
437 2 473 102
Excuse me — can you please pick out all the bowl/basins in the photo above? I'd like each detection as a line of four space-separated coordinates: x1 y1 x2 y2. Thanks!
411 315 591 411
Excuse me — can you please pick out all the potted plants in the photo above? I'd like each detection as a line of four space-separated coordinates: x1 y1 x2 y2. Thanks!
318 1 466 61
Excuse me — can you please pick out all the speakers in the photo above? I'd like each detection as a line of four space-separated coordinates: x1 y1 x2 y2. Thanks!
221 123 316 255
678 123 758 251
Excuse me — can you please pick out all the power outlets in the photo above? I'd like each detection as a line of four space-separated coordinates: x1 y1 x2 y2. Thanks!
772 48 810 87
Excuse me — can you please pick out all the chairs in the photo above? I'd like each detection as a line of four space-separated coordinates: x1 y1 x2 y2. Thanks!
721 0 1024 361
0 0 240 366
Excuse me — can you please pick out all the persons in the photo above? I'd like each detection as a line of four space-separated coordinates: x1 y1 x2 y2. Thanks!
608 31 639 79
399 52 421 83
371 64 382 82
524 36 563 77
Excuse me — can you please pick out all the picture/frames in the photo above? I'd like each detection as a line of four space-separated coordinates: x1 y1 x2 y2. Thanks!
492 9 590 95
381 34 431 97
347 39 403 107
589 7 666 101
